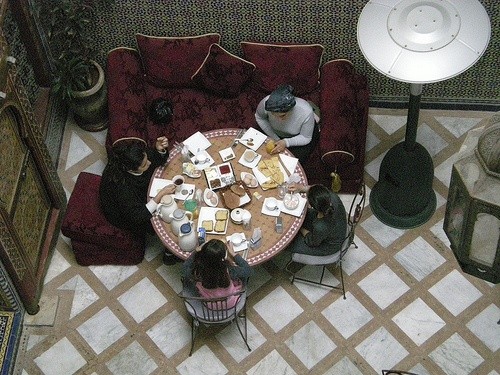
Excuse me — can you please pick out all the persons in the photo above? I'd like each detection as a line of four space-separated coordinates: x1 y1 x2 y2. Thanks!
285 183 347 256
255 88 320 165
183 239 253 311
99 136 182 264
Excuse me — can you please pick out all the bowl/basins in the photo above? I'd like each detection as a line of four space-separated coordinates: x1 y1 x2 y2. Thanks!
283 194 299 209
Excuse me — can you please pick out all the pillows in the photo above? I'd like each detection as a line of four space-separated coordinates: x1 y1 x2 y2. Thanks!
136 32 222 87
242 39 324 95
191 42 255 98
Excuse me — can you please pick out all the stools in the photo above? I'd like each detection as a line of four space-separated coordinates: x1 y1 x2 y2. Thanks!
61 171 145 268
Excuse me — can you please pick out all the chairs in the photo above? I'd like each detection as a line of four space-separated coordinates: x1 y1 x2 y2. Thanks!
287 182 366 300
176 276 252 356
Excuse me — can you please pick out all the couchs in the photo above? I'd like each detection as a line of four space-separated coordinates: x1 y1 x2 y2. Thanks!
106 47 371 196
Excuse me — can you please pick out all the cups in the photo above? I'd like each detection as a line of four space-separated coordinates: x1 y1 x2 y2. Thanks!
171 175 185 192
230 207 244 225
266 197 278 210
196 155 210 163
231 234 246 246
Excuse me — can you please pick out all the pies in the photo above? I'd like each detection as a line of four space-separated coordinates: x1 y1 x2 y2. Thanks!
224 185 246 209
258 157 284 188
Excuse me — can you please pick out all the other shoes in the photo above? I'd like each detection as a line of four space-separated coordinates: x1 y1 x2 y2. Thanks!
163 253 183 265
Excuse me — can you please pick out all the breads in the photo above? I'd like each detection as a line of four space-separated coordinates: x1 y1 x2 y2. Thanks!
202 220 213 232
186 164 194 173
188 208 200 220
214 220 226 232
215 210 228 221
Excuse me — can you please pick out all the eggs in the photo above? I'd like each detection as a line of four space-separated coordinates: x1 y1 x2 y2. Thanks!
243 174 257 186
207 191 217 204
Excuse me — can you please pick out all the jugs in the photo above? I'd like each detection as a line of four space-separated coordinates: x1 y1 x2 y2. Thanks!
169 208 193 237
178 221 197 252
155 194 178 224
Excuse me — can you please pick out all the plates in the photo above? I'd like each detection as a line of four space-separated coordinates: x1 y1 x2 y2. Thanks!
261 197 282 217
240 127 268 151
219 147 236 162
238 149 262 169
190 151 215 171
280 193 307 217
183 131 212 156
252 161 289 191
197 207 229 234
226 232 248 253
149 177 195 201
240 172 258 188
183 162 202 179
203 163 236 191
204 188 218 208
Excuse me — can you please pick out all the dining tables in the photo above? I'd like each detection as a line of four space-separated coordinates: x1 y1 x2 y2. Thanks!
148 128 310 267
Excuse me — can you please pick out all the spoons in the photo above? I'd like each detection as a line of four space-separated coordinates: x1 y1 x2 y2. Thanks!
181 189 193 206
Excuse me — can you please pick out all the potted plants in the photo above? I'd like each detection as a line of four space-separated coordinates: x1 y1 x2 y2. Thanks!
40 0 110 132
